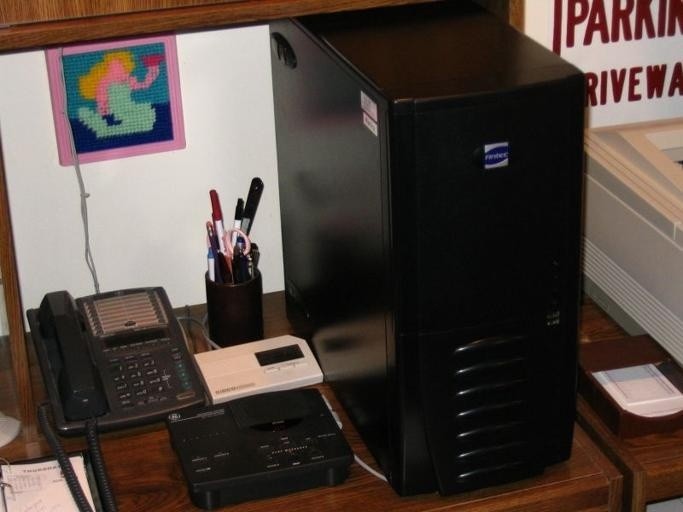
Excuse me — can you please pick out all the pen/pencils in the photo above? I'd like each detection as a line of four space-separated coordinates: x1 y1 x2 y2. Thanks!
251 243 260 274
247 253 254 277
215 253 225 282
240 253 252 282
210 190 232 274
232 246 244 284
232 197 245 249
208 246 216 281
240 178 264 236
206 220 217 245
237 236 245 254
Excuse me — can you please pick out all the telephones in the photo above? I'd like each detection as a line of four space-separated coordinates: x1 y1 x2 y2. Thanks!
26 285 206 436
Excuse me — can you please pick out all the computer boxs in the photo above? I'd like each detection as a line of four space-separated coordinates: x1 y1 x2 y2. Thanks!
268 11 589 496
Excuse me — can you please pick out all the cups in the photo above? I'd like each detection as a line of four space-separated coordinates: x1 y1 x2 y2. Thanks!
204 269 264 348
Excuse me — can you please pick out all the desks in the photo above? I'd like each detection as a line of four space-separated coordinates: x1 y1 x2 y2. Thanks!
0 291 623 512
575 287 682 511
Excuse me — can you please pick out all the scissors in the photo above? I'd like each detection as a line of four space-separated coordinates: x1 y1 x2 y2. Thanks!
207 228 253 283
